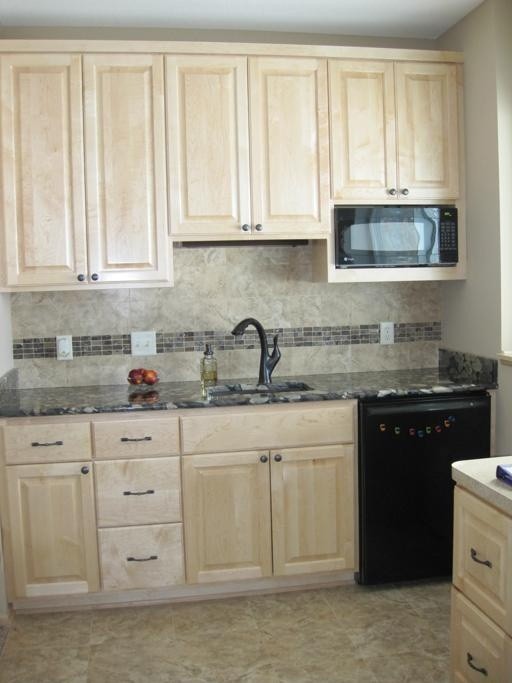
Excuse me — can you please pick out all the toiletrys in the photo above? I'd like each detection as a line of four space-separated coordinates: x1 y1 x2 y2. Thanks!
200 343 218 387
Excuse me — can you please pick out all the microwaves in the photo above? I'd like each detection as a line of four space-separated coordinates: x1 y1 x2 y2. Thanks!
333 201 458 268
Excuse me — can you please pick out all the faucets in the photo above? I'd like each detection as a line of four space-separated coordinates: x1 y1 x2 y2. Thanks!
230 317 281 384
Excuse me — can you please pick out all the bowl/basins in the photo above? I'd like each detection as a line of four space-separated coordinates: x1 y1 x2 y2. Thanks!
126 375 161 391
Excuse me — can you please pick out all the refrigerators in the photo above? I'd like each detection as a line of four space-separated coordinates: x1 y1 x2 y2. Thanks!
358 394 491 586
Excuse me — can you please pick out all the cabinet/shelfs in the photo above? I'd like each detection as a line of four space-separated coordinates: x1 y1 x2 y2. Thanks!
181 399 358 588
89 417 188 592
165 53 329 241
327 56 463 203
1 50 175 293
448 484 512 682
1 415 99 601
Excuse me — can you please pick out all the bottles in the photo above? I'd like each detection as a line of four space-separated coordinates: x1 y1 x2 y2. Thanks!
200 343 218 397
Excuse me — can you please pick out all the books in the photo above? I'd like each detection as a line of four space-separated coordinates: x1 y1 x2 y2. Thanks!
495 462 511 486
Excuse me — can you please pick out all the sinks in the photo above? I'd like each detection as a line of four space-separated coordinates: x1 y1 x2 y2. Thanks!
266 381 317 393
207 383 274 402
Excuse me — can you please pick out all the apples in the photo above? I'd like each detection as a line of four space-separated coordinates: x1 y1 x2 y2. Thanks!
129 368 157 385
128 389 160 404
